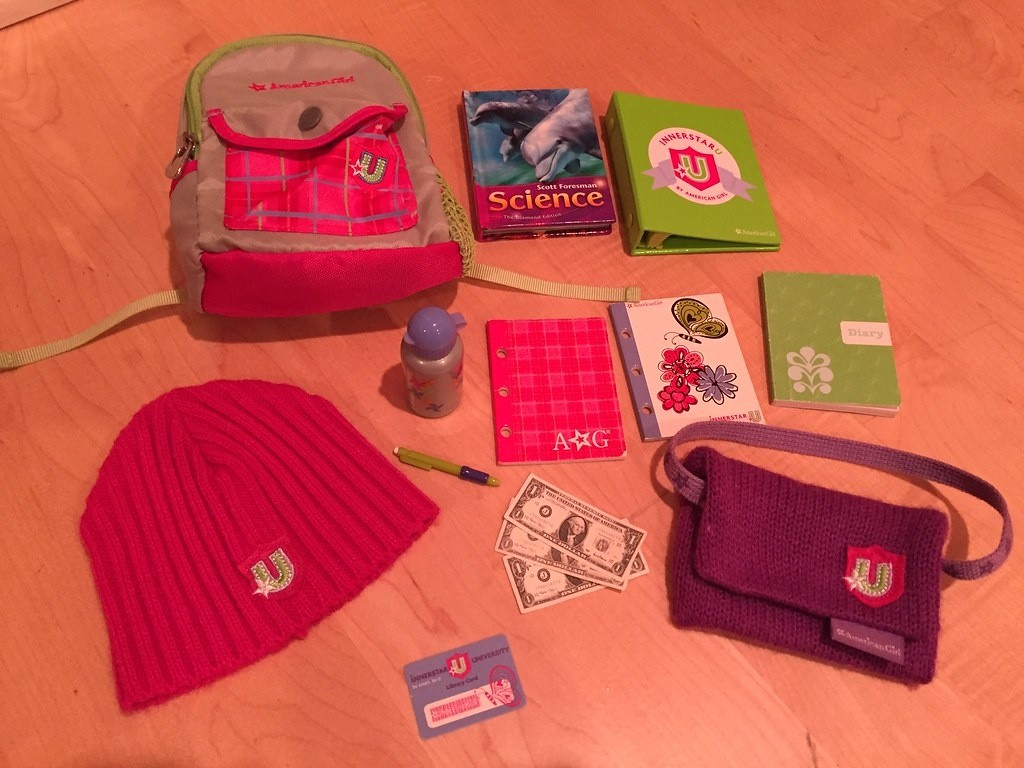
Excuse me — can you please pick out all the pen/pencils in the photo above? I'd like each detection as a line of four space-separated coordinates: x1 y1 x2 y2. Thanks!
393 445 499 490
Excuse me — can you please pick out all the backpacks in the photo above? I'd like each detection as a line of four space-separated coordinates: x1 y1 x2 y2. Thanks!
163 33 475 320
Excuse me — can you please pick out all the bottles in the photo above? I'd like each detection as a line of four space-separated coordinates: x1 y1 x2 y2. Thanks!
401 306 468 419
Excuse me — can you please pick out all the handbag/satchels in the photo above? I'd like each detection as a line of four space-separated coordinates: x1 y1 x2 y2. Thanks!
664 419 1012 683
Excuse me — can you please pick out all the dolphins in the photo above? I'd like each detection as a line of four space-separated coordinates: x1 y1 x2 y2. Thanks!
469 88 604 183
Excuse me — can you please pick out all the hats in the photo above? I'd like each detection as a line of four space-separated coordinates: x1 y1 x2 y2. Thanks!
75 376 440 710
